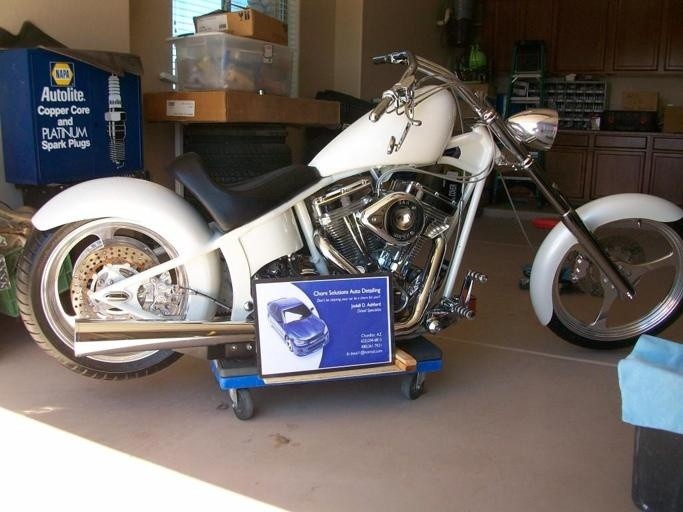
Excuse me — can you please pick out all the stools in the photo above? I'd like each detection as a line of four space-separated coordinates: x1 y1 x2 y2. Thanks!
491 142 545 210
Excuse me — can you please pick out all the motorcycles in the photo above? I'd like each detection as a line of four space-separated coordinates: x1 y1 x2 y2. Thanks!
17 48 683 381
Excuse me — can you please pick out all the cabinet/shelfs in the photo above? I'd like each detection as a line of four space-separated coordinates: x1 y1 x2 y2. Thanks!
527 81 608 130
480 0 682 78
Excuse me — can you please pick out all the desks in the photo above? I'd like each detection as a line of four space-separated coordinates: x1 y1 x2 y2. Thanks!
141 90 341 199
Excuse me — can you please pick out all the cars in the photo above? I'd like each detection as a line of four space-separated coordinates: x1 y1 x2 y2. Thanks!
267 297 331 358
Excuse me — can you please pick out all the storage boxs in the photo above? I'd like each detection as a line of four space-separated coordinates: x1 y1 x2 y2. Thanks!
174 8 296 97
621 87 683 132
